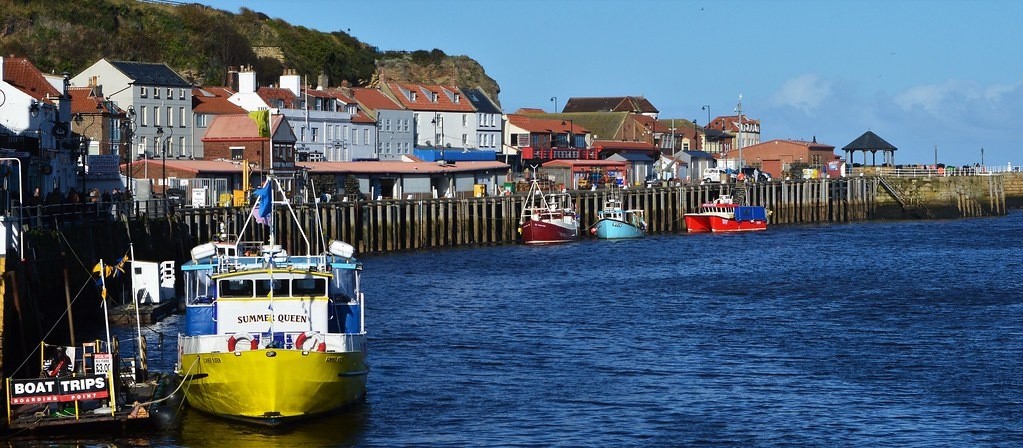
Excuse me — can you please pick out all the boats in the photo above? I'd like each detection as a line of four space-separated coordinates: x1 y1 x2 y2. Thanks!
6 244 162 443
175 109 369 427
684 194 767 232
519 164 579 246
587 182 647 240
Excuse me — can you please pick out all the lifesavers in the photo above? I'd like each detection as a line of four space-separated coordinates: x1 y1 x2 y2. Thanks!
296 330 326 353
227 332 257 352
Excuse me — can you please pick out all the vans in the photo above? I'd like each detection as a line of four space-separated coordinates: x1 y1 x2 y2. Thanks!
703 168 726 183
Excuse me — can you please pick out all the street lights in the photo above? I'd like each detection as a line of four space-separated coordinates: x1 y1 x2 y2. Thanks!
561 120 572 147
29 101 52 204
156 126 173 213
702 105 710 129
551 97 557 113
431 117 444 161
74 113 94 224
734 93 742 173
119 105 137 220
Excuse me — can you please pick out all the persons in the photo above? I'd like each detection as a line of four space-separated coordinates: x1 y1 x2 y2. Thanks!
48 344 75 416
29 186 134 221
319 189 327 202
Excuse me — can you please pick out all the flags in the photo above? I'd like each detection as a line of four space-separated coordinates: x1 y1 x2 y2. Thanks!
253 181 272 217
247 110 270 138
252 206 271 226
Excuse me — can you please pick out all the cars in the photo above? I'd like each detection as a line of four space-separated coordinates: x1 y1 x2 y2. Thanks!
733 168 772 182
